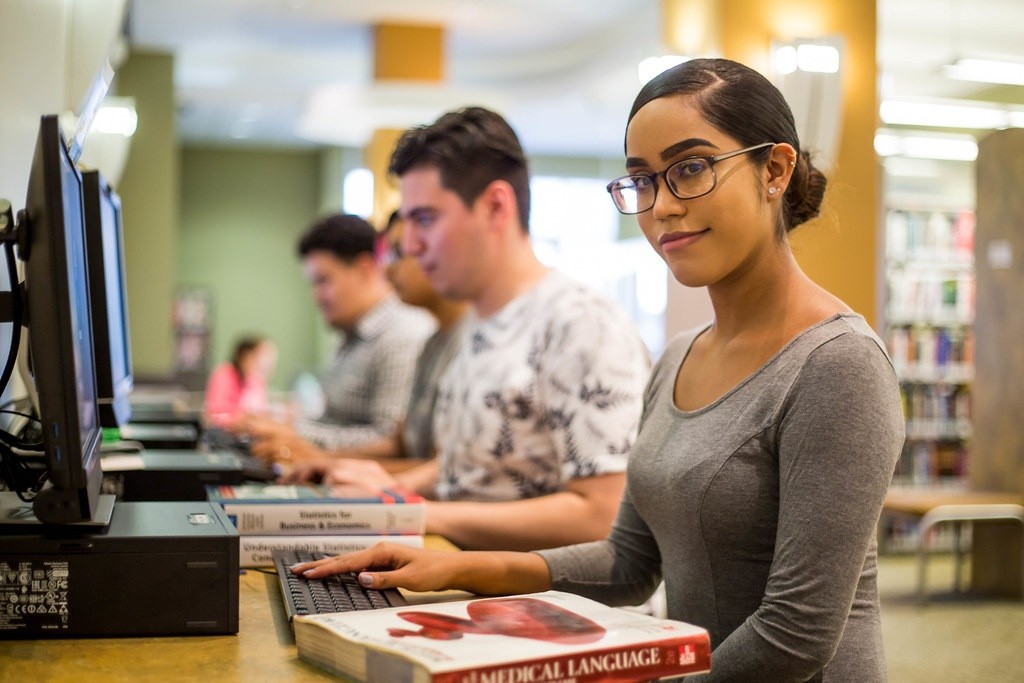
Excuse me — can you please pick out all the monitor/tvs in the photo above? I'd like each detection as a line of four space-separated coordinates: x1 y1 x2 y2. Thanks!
80 169 133 430
23 112 116 531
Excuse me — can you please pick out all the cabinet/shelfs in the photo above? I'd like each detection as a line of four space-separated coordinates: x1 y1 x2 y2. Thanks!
876 123 1024 600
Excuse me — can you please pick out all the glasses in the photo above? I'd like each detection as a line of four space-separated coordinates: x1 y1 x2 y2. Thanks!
606 142 777 216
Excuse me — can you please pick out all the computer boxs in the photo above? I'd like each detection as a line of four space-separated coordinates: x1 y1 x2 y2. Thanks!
0 404 244 641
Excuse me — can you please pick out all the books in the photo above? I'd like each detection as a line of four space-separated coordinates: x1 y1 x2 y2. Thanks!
289 591 713 682
238 536 425 567
868 139 983 552
207 483 434 536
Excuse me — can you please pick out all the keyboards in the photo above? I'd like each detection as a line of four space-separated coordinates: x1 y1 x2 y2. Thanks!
271 548 409 623
205 425 282 483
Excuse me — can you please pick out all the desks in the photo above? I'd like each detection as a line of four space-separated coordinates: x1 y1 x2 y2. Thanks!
0 461 494 683
879 487 1024 610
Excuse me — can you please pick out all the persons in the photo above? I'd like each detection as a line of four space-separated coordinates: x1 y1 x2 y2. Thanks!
290 59 909 683
202 105 652 551
